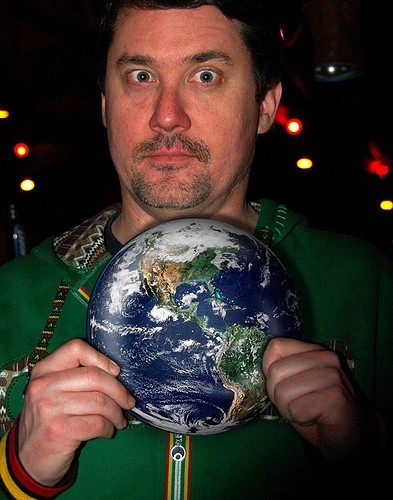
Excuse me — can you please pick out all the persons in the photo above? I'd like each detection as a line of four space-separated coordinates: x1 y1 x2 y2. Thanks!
1 0 393 500
